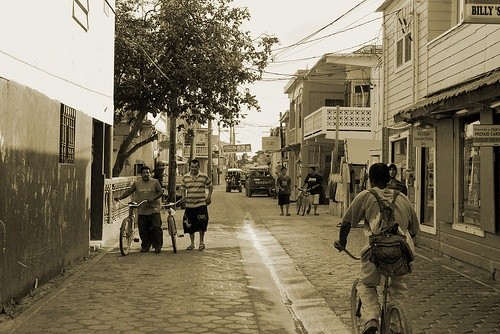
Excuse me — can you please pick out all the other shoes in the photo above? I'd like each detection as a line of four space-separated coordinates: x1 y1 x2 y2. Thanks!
154 248 162 253
187 245 195 250
140 247 150 252
314 213 320 215
198 244 205 249
286 213 290 216
306 209 310 214
361 320 378 334
279 213 284 216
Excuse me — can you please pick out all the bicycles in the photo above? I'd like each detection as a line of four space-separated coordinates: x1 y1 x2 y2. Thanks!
293 184 312 216
161 197 188 253
113 198 148 255
335 242 409 334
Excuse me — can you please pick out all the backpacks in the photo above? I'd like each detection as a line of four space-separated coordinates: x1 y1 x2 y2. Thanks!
368 187 414 277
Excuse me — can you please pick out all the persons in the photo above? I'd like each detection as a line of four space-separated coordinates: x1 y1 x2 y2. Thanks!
334 162 421 334
275 166 292 216
386 164 408 197
180 159 213 250
300 166 323 215
114 166 163 253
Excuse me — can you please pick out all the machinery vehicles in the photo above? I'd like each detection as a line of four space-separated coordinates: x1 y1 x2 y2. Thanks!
225 168 244 193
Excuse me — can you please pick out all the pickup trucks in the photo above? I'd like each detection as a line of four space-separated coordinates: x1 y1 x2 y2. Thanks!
242 169 275 197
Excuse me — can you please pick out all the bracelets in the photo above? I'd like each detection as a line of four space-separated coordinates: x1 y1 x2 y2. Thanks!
311 187 312 190
